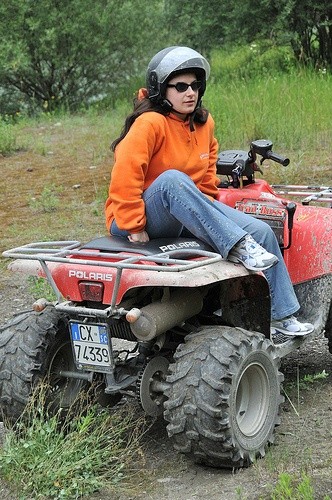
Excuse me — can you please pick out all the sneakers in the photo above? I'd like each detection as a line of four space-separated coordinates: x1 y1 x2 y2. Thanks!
227 233 279 271
270 315 314 337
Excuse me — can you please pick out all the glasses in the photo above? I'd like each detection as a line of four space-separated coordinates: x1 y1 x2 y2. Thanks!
167 80 203 92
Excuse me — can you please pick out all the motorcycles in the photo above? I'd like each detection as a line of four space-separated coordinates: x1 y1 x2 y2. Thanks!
0 137 331 473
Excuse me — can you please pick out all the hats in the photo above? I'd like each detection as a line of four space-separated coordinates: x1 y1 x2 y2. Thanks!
145 46 210 107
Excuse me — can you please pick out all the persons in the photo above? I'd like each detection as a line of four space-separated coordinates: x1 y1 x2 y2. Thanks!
105 46 314 335
